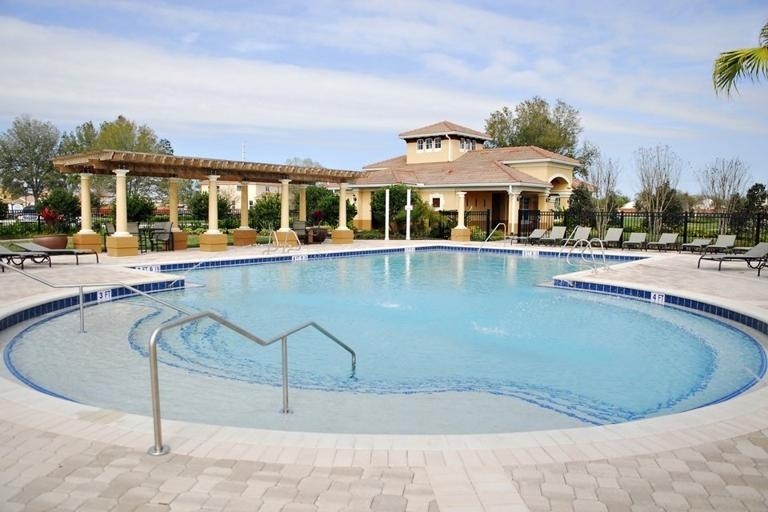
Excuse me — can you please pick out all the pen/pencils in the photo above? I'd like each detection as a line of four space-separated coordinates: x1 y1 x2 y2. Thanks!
7 204 24 219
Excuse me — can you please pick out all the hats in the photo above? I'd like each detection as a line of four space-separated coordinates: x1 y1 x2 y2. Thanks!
16 205 46 222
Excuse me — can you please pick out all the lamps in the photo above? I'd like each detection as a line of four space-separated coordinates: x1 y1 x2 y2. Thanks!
505 226 768 277
291 219 323 244
0 242 99 272
104 221 173 254
